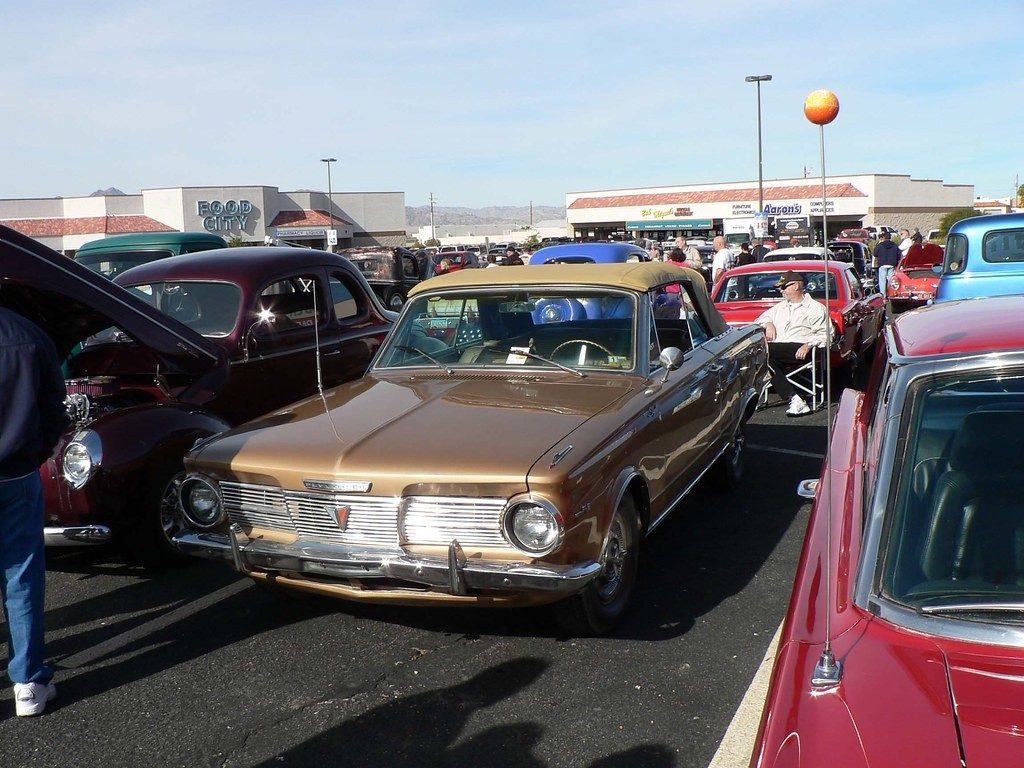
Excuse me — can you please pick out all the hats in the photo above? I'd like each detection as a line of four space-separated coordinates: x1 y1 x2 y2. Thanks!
773 270 805 287
505 246 515 252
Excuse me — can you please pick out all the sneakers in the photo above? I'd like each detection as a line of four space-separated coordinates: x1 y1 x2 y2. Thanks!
12 682 56 715
785 394 810 416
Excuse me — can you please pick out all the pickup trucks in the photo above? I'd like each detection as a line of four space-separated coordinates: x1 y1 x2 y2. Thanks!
835 226 900 245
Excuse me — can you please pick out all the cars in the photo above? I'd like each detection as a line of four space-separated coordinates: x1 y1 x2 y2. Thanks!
927 212 1024 306
887 242 945 314
159 260 772 631
827 242 872 275
923 229 946 249
0 224 447 547
762 247 836 261
752 295 1024 768
60 232 387 382
712 261 886 391
335 237 777 314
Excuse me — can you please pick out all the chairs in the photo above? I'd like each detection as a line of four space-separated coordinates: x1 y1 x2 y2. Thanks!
919 408 1024 597
751 317 841 412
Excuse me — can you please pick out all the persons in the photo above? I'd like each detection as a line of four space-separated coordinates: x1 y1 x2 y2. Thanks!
863 227 872 239
898 228 923 257
755 270 835 417
633 235 830 311
873 231 902 305
0 304 68 717
433 246 536 277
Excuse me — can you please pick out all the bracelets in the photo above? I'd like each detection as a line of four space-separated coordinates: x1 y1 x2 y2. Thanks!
806 342 813 348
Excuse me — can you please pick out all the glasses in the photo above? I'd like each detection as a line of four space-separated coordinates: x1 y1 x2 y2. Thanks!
781 283 794 290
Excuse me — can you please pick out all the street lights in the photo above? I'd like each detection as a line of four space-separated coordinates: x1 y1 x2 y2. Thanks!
321 158 337 254
746 75 773 246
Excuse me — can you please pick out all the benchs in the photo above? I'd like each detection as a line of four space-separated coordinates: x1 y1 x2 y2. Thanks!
467 326 684 362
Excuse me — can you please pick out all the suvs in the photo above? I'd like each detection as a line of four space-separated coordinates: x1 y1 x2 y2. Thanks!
526 242 683 324
